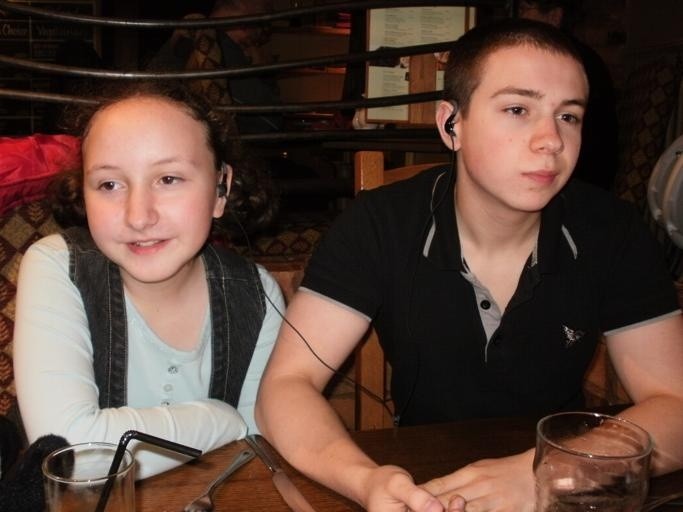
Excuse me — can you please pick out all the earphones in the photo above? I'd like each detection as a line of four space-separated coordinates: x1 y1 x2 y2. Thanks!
444 113 456 137
217 162 228 198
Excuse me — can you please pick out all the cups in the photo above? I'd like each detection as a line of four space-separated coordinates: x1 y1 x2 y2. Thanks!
533 410 651 512
42 441 135 512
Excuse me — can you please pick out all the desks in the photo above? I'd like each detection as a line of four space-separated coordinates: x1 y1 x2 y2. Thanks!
46 401 680 511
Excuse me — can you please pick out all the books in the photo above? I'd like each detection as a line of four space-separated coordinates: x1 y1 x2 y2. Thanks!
365 8 478 123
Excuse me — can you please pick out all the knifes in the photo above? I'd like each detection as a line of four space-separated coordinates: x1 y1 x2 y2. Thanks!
245 429 317 511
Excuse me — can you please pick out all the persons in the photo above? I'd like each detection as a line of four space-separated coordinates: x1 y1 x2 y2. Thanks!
11 83 286 489
255 18 683 512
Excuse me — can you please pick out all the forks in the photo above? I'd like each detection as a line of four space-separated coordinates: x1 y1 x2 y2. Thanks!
183 444 256 511
